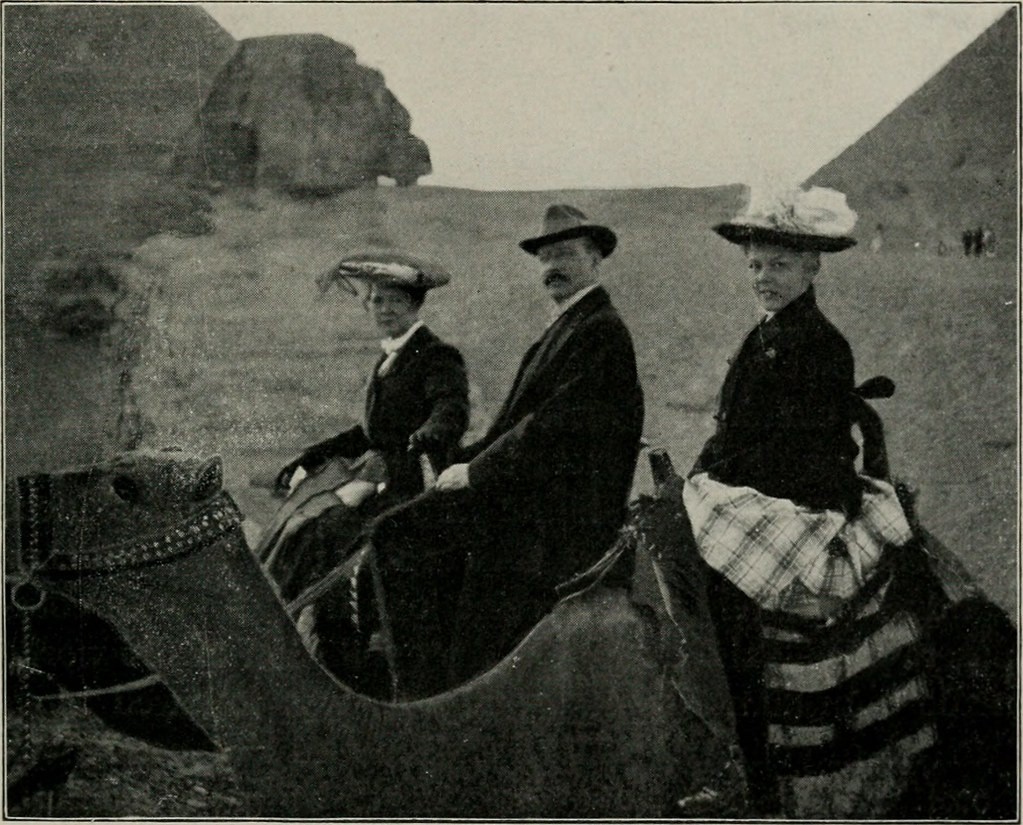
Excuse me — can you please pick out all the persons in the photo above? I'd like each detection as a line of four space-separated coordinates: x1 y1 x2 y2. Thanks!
349 205 647 701
676 183 875 812
277 237 470 677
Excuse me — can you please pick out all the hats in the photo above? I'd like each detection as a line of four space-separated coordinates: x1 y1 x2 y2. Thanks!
709 172 858 252
315 239 452 298
517 203 618 259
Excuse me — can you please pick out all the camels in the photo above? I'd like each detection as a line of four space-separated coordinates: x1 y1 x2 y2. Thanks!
2 445 1016 821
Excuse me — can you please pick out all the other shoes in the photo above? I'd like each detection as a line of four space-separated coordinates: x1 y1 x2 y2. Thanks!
660 789 731 819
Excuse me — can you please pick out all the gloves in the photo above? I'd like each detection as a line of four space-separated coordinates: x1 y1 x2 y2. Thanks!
273 454 307 495
405 425 447 454
